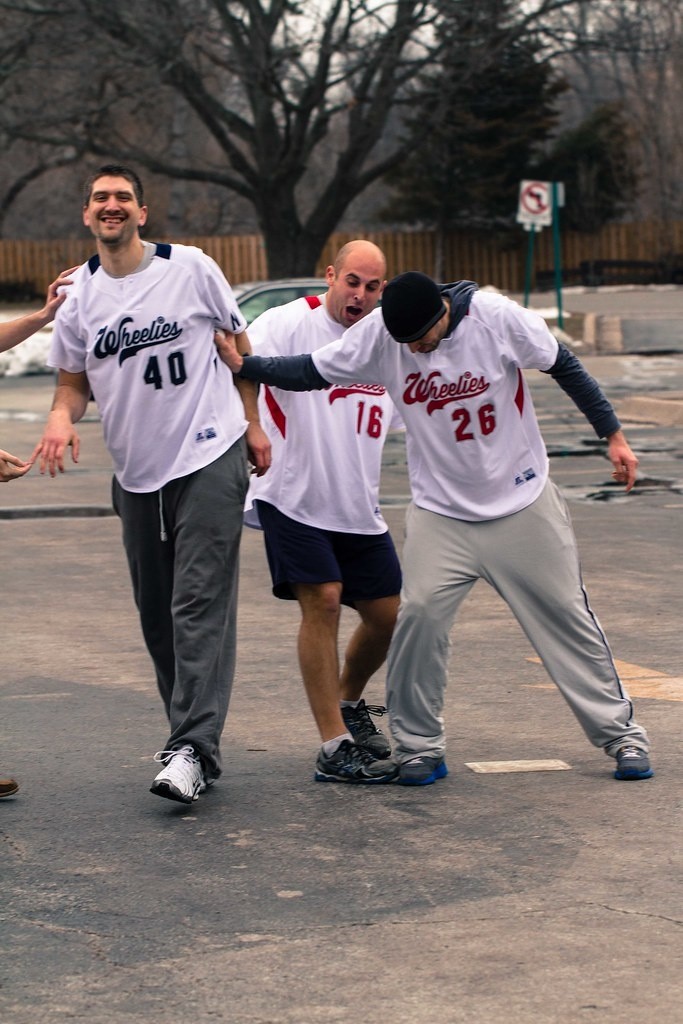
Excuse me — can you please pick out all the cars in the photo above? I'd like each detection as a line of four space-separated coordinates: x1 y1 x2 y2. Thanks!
223 277 386 330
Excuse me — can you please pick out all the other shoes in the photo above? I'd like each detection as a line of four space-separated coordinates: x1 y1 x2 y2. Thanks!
0 779 19 797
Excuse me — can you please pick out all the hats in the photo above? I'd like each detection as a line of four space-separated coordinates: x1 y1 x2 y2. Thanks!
381 271 447 344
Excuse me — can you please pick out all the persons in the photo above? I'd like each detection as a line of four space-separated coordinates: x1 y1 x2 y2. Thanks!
212 271 654 785
242 238 402 782
1 262 79 800
27 163 271 807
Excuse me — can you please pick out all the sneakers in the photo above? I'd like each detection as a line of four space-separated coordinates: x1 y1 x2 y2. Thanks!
340 699 392 760
149 744 215 804
614 745 654 780
399 755 449 786
314 739 402 785
